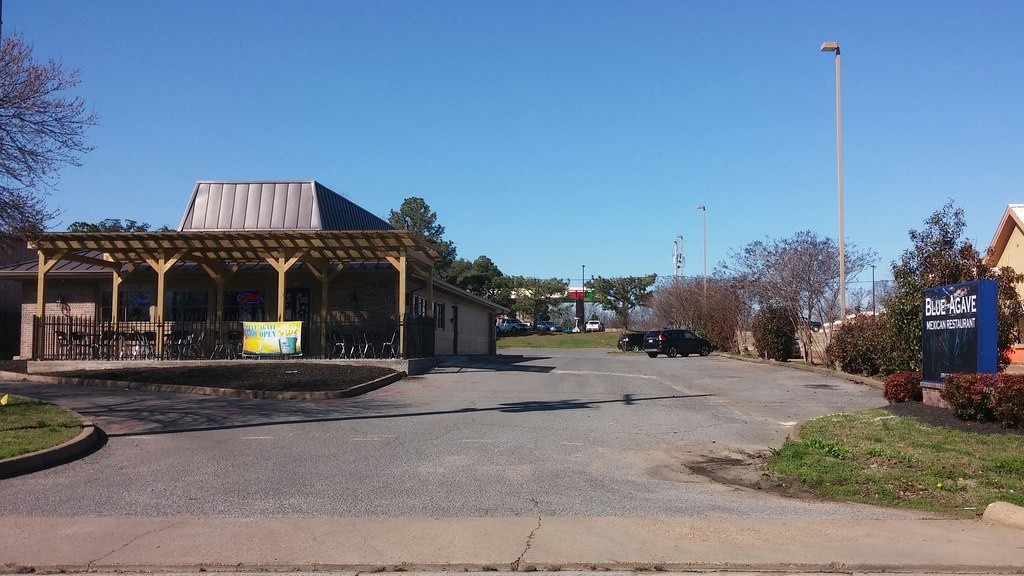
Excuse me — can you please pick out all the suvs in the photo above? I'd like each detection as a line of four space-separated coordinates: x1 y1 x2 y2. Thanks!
796 317 822 333
496 317 528 333
644 327 712 358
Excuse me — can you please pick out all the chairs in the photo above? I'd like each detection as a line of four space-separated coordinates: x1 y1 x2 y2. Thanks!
327 329 400 360
54 329 243 360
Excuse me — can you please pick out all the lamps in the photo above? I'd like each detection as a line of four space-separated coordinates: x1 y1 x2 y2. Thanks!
56 293 63 303
351 292 357 303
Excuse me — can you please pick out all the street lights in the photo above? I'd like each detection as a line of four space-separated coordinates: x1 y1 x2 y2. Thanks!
820 42 848 324
870 265 877 317
697 204 708 300
581 264 586 296
675 234 685 282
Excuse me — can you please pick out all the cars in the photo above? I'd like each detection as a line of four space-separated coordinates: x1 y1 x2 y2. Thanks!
585 320 606 333
536 321 564 332
616 332 644 353
